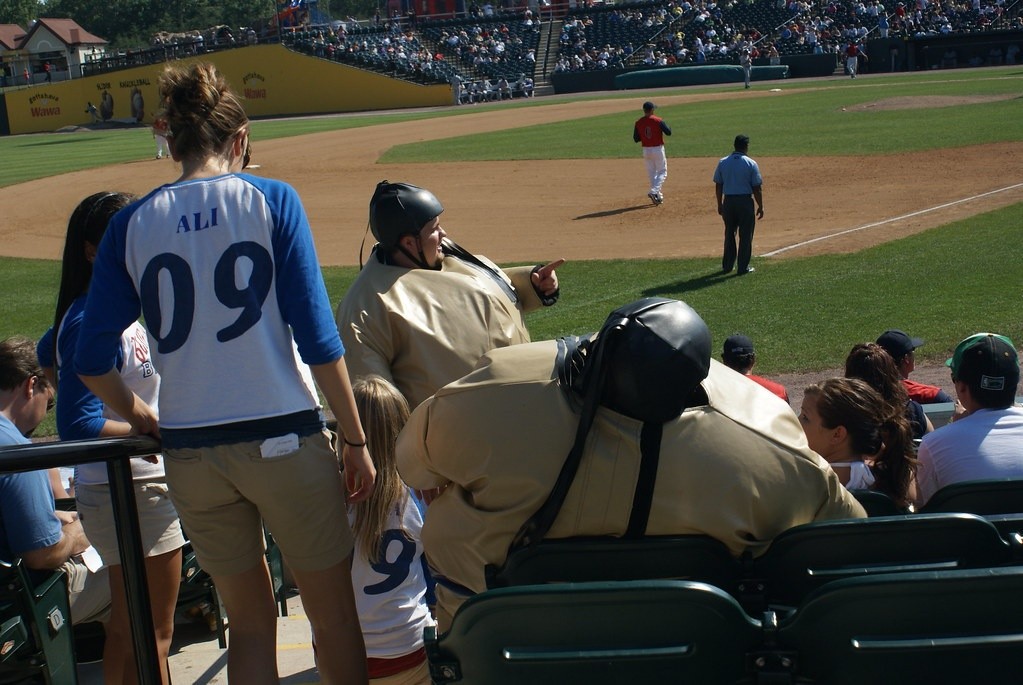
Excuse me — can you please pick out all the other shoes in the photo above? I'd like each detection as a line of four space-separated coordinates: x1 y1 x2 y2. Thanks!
748 267 755 273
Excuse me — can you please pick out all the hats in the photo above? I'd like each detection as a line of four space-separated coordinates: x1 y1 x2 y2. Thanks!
876 330 922 358
643 102 657 112
369 179 444 243
579 296 713 424
724 334 754 356
944 332 1020 393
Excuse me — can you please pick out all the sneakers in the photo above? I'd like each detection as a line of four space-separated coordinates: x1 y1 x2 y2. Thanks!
648 192 663 206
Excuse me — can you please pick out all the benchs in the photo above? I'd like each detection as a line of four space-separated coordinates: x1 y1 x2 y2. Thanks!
0 497 288 685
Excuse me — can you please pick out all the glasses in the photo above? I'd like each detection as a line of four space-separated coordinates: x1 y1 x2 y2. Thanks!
28 367 57 410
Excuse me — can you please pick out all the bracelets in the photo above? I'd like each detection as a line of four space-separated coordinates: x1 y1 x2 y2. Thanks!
344 437 366 447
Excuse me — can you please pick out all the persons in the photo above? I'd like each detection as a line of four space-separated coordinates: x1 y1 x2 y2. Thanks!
0 183 1023 685
0 0 1023 160
713 134 764 275
633 102 671 206
72 63 376 685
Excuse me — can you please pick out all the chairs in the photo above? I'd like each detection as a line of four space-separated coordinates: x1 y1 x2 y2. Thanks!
423 402 1023 685
